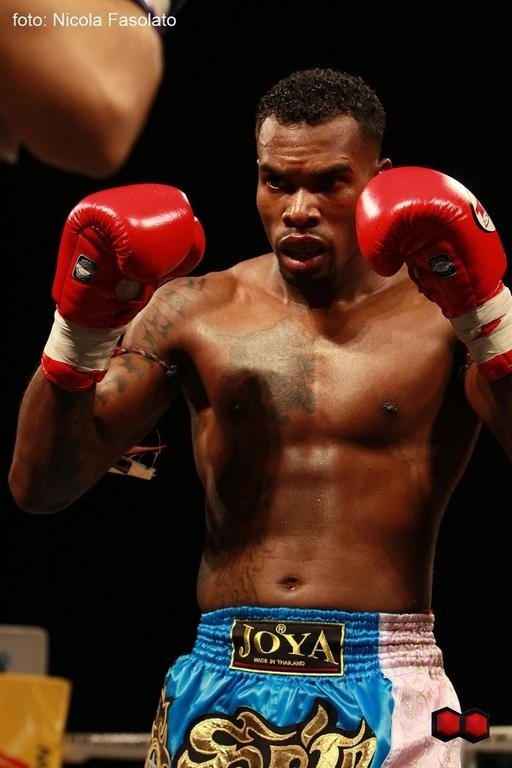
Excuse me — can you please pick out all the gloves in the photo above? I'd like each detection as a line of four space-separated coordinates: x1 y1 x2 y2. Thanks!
41 183 205 392
356 167 512 380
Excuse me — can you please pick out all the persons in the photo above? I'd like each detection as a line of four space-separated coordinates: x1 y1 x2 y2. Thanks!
8 66 512 768
0 0 188 179
